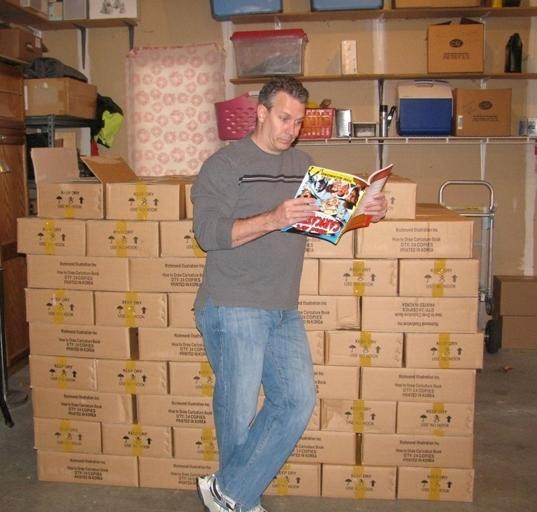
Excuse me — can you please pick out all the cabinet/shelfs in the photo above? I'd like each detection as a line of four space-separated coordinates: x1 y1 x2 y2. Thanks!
226 4 535 149
23 113 105 216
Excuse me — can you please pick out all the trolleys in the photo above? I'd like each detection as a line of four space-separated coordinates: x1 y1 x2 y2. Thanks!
437 180 499 353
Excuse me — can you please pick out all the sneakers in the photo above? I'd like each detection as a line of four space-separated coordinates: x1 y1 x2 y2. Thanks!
195 473 267 512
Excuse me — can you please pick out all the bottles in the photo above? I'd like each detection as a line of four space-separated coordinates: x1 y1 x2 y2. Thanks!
379 104 386 136
504 33 523 73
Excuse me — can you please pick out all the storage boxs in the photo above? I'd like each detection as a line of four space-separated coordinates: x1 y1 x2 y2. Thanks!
229 27 308 78
17 147 485 503
424 18 488 77
489 273 536 318
451 83 514 138
391 1 483 12
309 2 385 12
0 0 137 119
497 318 536 351
396 80 453 138
210 1 283 23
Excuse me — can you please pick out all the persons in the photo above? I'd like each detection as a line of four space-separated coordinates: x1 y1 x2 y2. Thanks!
192 73 388 511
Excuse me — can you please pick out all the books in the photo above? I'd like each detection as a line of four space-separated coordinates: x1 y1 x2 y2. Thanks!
281 162 394 246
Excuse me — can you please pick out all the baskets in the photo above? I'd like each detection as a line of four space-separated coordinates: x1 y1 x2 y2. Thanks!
296 107 336 139
213 90 260 140
